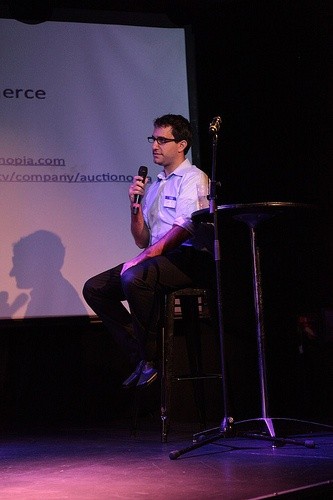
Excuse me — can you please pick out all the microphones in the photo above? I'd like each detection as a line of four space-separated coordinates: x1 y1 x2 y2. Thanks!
131 165 149 214
210 115 222 134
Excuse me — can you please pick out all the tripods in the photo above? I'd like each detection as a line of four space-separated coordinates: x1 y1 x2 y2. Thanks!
166 127 318 459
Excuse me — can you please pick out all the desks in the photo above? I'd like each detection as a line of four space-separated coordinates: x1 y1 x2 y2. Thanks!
167 200 333 458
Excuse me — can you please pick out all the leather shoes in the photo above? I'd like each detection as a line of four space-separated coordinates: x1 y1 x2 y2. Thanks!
122 362 144 389
136 360 158 386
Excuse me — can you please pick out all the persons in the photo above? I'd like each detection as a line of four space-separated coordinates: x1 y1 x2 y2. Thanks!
83 113 211 385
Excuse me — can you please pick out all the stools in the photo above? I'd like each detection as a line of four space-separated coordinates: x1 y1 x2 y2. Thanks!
131 284 228 443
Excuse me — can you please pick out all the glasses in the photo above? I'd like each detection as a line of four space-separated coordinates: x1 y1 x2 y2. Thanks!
147 136 180 146
